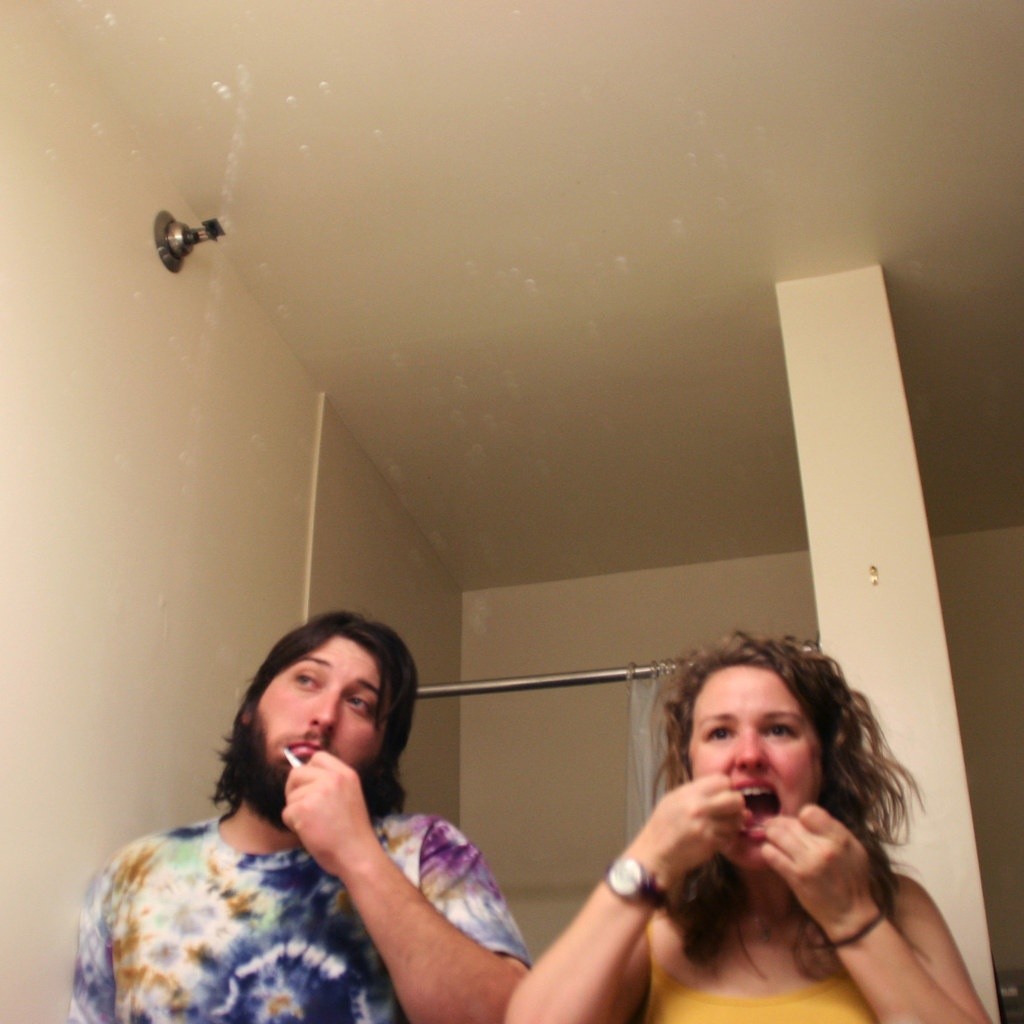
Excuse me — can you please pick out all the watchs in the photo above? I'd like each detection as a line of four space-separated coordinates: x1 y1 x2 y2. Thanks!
605 859 665 903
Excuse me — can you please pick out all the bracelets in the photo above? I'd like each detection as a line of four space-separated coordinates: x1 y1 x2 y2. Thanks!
835 911 884 947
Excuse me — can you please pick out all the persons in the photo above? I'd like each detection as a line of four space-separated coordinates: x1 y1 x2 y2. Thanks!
505 631 994 1024
66 611 530 1024
